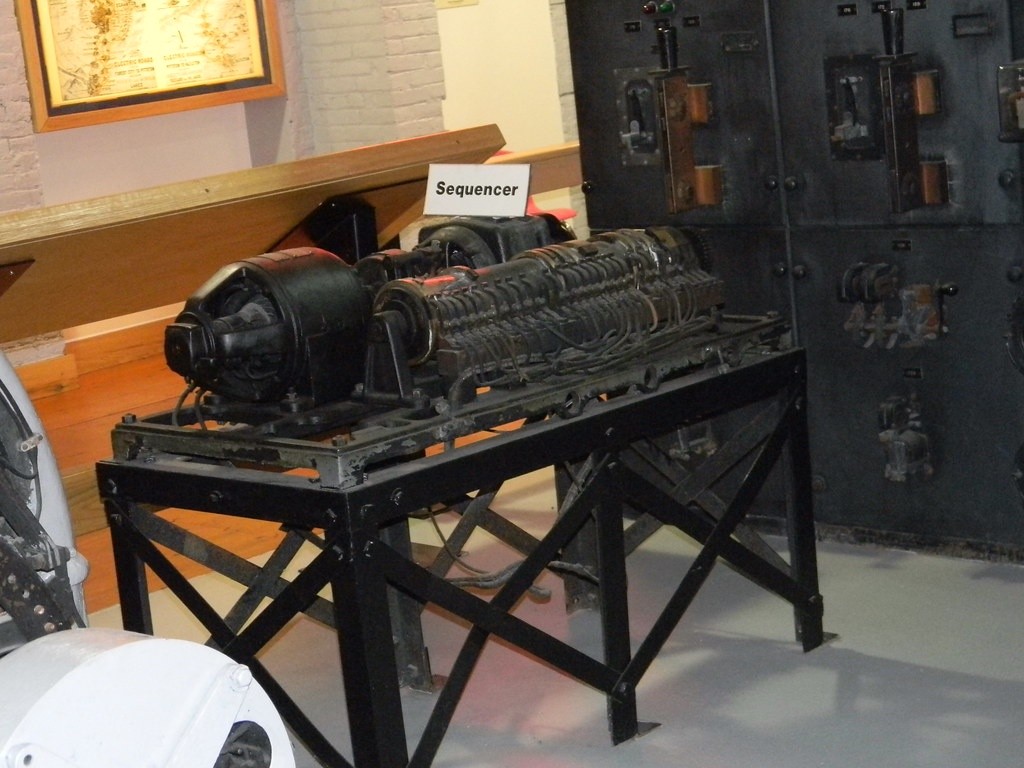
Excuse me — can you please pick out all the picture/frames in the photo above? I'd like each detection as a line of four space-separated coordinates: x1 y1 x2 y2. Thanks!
14 0 287 136
